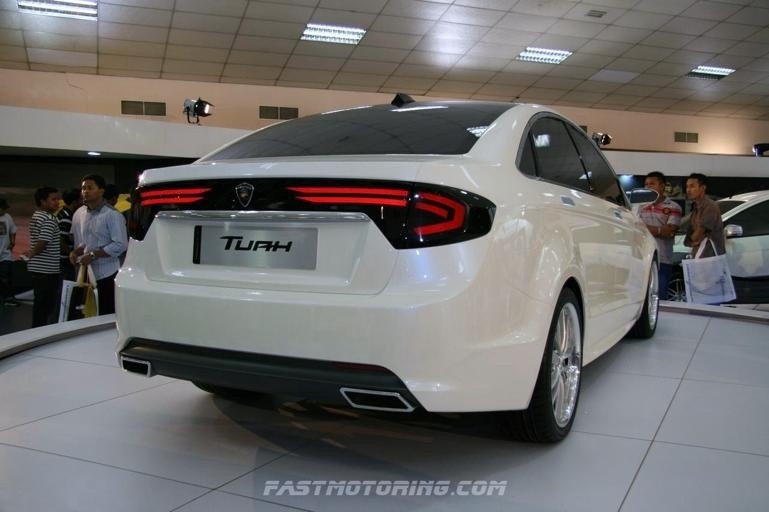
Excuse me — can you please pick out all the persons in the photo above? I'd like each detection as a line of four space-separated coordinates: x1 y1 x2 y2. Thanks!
26 173 128 328
639 171 683 301
682 173 724 306
0 199 20 307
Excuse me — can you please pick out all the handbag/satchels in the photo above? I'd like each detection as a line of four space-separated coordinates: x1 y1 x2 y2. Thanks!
58 264 101 322
681 237 737 306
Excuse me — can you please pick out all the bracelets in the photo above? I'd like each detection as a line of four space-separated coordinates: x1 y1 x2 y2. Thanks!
657 227 661 237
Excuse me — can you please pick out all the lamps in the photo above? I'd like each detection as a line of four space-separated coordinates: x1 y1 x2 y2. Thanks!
183 97 214 123
753 143 769 156
593 130 612 148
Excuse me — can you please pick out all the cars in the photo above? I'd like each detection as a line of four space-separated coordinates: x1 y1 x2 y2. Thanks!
666 188 769 303
114 91 661 445
